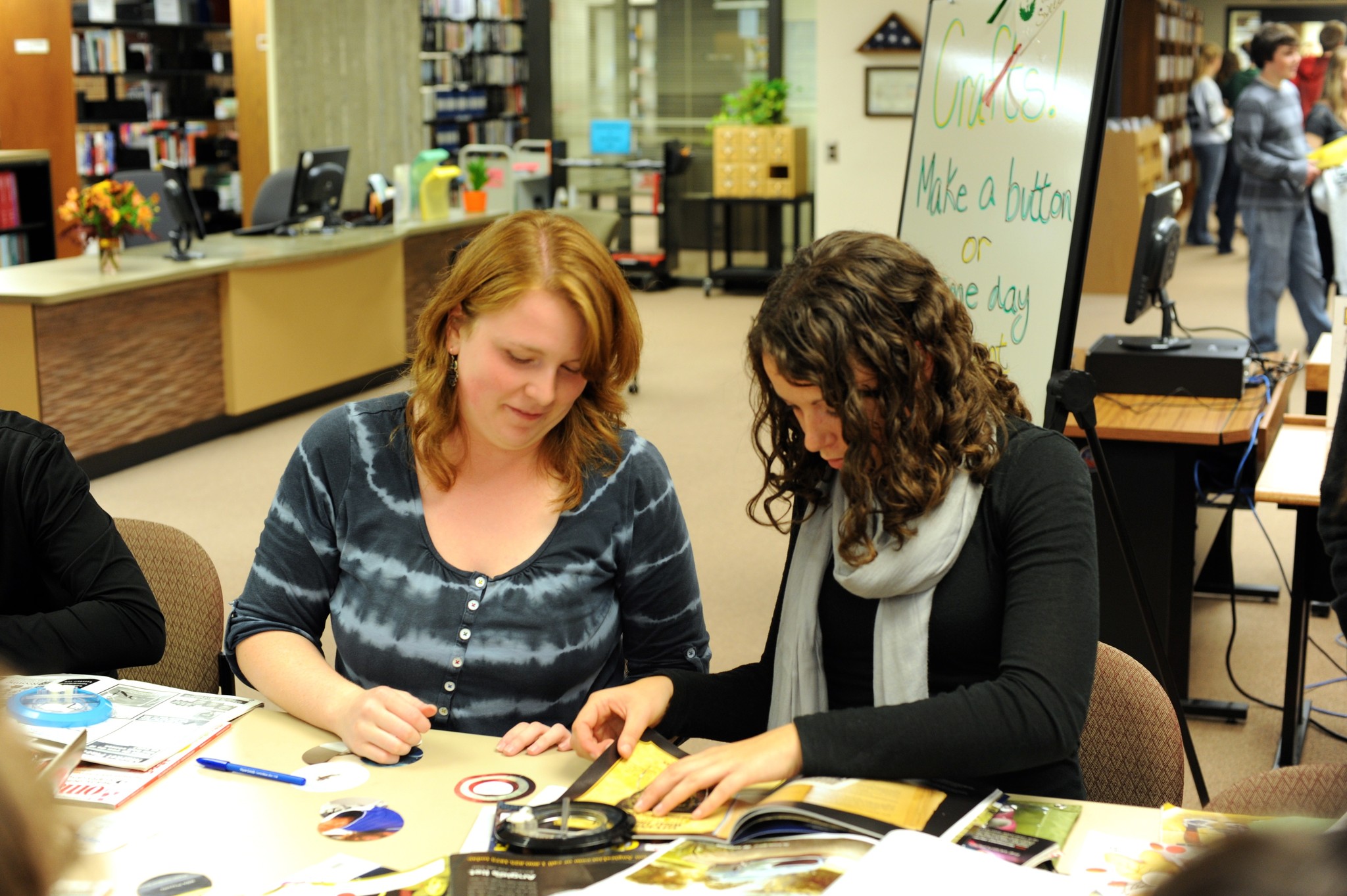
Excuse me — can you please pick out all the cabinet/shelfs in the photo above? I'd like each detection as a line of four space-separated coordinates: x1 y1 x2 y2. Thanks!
1082 116 1163 295
69 0 244 236
419 0 529 192
1121 0 1206 219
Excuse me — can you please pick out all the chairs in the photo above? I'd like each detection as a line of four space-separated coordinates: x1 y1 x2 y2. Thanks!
1078 641 1184 809
251 166 297 226
111 518 237 697
110 168 181 249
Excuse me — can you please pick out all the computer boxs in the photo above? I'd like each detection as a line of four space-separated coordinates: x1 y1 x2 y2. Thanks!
1083 334 1252 400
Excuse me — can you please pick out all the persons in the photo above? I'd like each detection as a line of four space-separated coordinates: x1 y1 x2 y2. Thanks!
0 408 168 680
221 210 712 769
1188 19 1347 355
567 231 1100 820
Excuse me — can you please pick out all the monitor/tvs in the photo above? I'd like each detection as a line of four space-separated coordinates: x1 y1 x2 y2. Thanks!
1118 180 1192 352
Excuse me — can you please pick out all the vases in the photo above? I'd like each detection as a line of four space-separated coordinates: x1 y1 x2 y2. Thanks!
99 237 120 275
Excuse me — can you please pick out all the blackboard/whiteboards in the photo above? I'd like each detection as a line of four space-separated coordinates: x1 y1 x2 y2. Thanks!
896 0 1123 433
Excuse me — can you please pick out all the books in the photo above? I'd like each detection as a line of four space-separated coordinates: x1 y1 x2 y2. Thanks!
956 827 1061 870
72 29 127 74
557 727 966 846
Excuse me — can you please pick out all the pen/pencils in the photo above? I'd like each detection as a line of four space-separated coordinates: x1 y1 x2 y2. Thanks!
196 758 305 785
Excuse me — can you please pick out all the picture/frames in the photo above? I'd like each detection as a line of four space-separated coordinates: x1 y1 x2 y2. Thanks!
865 65 919 118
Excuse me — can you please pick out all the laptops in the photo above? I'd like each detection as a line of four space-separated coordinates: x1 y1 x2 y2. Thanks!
232 147 351 237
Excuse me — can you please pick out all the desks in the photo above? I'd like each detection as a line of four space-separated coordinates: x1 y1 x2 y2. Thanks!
0 673 1347 896
0 207 510 481
1063 331 1335 772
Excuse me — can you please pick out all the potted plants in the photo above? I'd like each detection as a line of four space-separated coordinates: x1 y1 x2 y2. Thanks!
462 154 488 212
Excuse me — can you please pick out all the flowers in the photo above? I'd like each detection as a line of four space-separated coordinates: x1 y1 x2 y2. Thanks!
56 179 162 274
